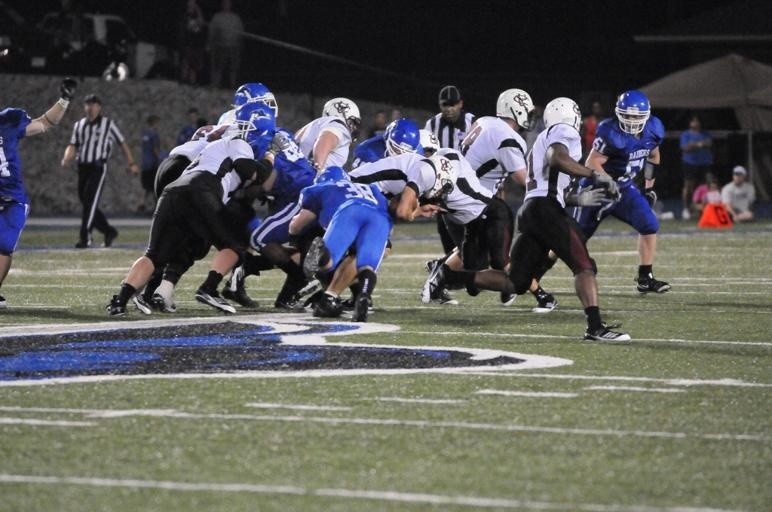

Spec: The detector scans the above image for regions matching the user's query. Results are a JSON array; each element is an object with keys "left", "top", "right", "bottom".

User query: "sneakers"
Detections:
[
  {"left": 421, "top": 263, "right": 448, "bottom": 305},
  {"left": 302, "top": 236, "right": 324, "bottom": 277},
  {"left": 584, "top": 321, "right": 632, "bottom": 342},
  {"left": 500, "top": 291, "right": 516, "bottom": 306},
  {"left": 100, "top": 226, "right": 118, "bottom": 248},
  {"left": 433, "top": 289, "right": 458, "bottom": 306},
  {"left": 532, "top": 293, "right": 559, "bottom": 313},
  {"left": 75, "top": 239, "right": 92, "bottom": 248},
  {"left": 106, "top": 271, "right": 379, "bottom": 322},
  {"left": 636, "top": 272, "right": 670, "bottom": 294}
]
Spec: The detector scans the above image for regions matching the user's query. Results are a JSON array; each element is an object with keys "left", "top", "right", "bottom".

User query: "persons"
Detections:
[
  {"left": 679, "top": 115, "right": 717, "bottom": 220},
  {"left": 107, "top": 82, "right": 671, "bottom": 342},
  {"left": 691, "top": 171, "right": 721, "bottom": 216},
  {"left": 721, "top": 165, "right": 759, "bottom": 222},
  {"left": 0, "top": 77, "right": 77, "bottom": 309},
  {"left": 60, "top": 93, "right": 139, "bottom": 248}
]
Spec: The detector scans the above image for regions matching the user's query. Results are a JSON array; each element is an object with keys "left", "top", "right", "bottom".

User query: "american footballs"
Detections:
[{"left": 388, "top": 194, "right": 418, "bottom": 219}]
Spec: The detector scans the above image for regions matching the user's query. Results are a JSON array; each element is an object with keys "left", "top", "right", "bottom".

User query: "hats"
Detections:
[
  {"left": 439, "top": 84, "right": 461, "bottom": 106},
  {"left": 733, "top": 165, "right": 746, "bottom": 177},
  {"left": 84, "top": 93, "right": 101, "bottom": 104}
]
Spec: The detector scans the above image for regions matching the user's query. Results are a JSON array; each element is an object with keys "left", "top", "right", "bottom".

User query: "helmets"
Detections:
[
  {"left": 496, "top": 87, "right": 538, "bottom": 132},
  {"left": 228, "top": 82, "right": 280, "bottom": 162},
  {"left": 383, "top": 117, "right": 421, "bottom": 158},
  {"left": 422, "top": 155, "right": 458, "bottom": 205},
  {"left": 614, "top": 90, "right": 651, "bottom": 134},
  {"left": 322, "top": 97, "right": 362, "bottom": 134},
  {"left": 543, "top": 95, "right": 582, "bottom": 131},
  {"left": 419, "top": 128, "right": 440, "bottom": 150}
]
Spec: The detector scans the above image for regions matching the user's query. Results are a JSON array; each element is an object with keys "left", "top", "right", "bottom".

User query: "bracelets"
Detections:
[{"left": 58, "top": 98, "right": 71, "bottom": 110}]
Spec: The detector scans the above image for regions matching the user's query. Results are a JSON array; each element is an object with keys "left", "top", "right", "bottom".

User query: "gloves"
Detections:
[
  {"left": 589, "top": 170, "right": 617, "bottom": 198},
  {"left": 256, "top": 184, "right": 280, "bottom": 206},
  {"left": 576, "top": 184, "right": 607, "bottom": 209},
  {"left": 60, "top": 77, "right": 79, "bottom": 102},
  {"left": 268, "top": 132, "right": 290, "bottom": 155},
  {"left": 643, "top": 187, "right": 658, "bottom": 208}
]
